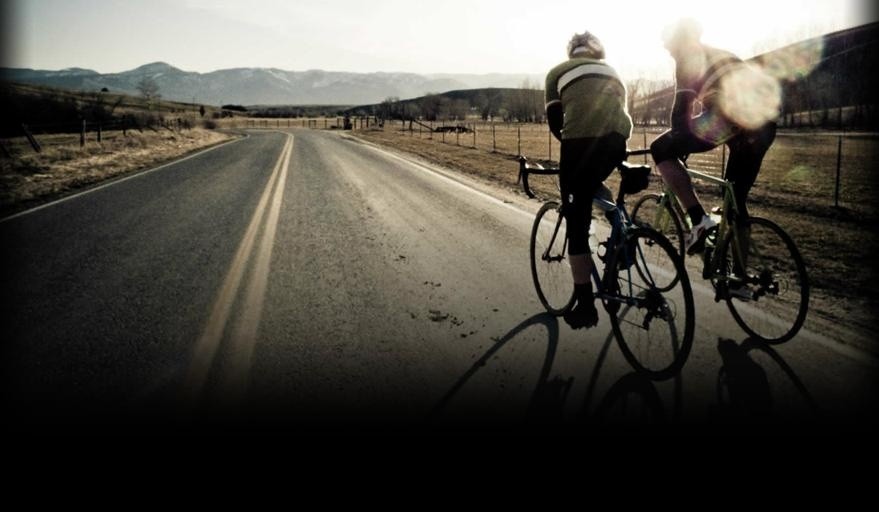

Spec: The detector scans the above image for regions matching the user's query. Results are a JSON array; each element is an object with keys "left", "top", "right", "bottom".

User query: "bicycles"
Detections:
[
  {"left": 630, "top": 148, "right": 810, "bottom": 345},
  {"left": 514, "top": 153, "right": 697, "bottom": 384}
]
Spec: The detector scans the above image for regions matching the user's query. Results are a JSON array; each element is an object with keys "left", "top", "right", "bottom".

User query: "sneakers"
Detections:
[
  {"left": 687, "top": 215, "right": 719, "bottom": 255},
  {"left": 615, "top": 219, "right": 638, "bottom": 270},
  {"left": 714, "top": 273, "right": 751, "bottom": 301},
  {"left": 563, "top": 305, "right": 598, "bottom": 327}
]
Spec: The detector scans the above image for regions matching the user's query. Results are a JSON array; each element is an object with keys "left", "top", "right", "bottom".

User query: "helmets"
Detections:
[{"left": 568, "top": 32, "right": 603, "bottom": 59}]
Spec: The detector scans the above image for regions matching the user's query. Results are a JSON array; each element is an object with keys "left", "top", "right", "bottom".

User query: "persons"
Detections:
[
  {"left": 649, "top": 26, "right": 777, "bottom": 290},
  {"left": 544, "top": 32, "right": 637, "bottom": 330}
]
